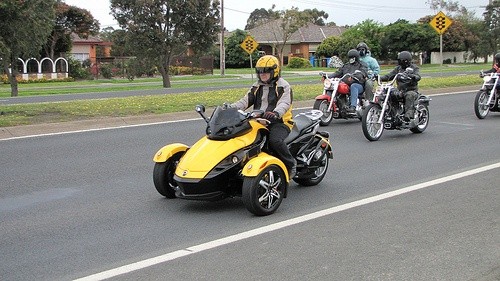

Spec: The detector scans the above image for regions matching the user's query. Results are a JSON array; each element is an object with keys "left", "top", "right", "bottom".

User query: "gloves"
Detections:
[{"left": 262, "top": 112, "right": 279, "bottom": 121}]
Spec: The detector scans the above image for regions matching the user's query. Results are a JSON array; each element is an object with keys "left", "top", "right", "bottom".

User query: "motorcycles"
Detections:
[
  {"left": 473, "top": 68, "right": 500, "bottom": 120},
  {"left": 313, "top": 69, "right": 374, "bottom": 127},
  {"left": 152, "top": 103, "right": 334, "bottom": 216},
  {"left": 362, "top": 67, "right": 433, "bottom": 141}
]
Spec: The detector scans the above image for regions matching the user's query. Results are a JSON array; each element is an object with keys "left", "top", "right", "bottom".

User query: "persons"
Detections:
[
  {"left": 222, "top": 55, "right": 297, "bottom": 179},
  {"left": 356, "top": 42, "right": 381, "bottom": 100},
  {"left": 373, "top": 51, "right": 421, "bottom": 122},
  {"left": 320, "top": 50, "right": 366, "bottom": 112},
  {"left": 479, "top": 53, "right": 500, "bottom": 95}
]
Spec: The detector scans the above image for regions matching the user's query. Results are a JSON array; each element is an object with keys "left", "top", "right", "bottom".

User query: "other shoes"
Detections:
[
  {"left": 350, "top": 106, "right": 356, "bottom": 112},
  {"left": 405, "top": 115, "right": 411, "bottom": 125},
  {"left": 289, "top": 158, "right": 298, "bottom": 180}
]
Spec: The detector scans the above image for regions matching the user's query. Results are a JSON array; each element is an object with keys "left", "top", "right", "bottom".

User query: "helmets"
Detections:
[
  {"left": 495, "top": 53, "right": 500, "bottom": 58},
  {"left": 357, "top": 43, "right": 368, "bottom": 49},
  {"left": 347, "top": 49, "right": 360, "bottom": 61},
  {"left": 255, "top": 55, "right": 280, "bottom": 84},
  {"left": 397, "top": 51, "right": 413, "bottom": 60}
]
